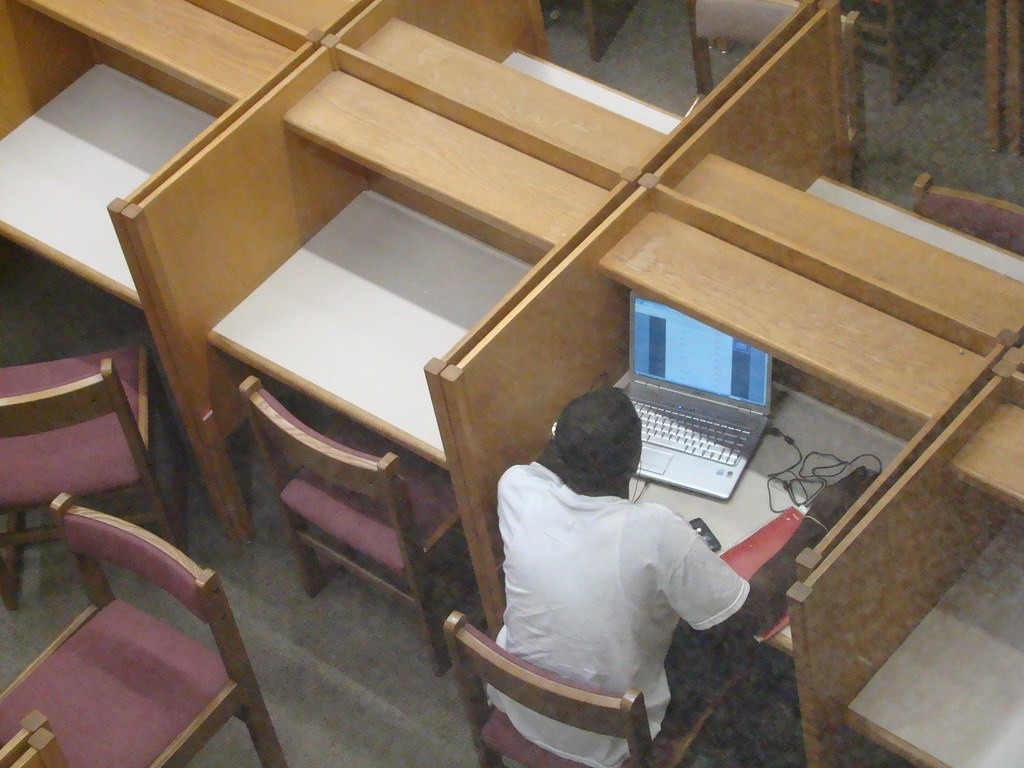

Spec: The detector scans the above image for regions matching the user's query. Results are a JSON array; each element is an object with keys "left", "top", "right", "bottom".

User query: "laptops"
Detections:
[{"left": 621, "top": 284, "right": 772, "bottom": 501}]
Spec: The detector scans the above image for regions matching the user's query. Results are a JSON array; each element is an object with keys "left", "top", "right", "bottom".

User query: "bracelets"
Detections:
[{"left": 805, "top": 516, "right": 828, "bottom": 533}]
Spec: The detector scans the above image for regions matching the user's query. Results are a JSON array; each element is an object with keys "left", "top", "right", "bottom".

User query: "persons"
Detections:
[{"left": 487, "top": 387, "right": 882, "bottom": 768}]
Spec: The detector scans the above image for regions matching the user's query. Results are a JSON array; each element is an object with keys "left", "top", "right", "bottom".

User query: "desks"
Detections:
[{"left": 0, "top": 47, "right": 1024, "bottom": 768}]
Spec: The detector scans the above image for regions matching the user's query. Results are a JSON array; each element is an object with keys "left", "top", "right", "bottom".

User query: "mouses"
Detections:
[{"left": 861, "top": 467, "right": 879, "bottom": 488}]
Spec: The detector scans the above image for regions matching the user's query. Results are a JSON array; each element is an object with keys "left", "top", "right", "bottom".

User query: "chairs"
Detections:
[
  {"left": 0, "top": 493, "right": 288, "bottom": 768},
  {"left": 443, "top": 610, "right": 772, "bottom": 768},
  {"left": 683, "top": 0, "right": 866, "bottom": 193},
  {"left": 239, "top": 373, "right": 458, "bottom": 678},
  {"left": 0, "top": 343, "right": 207, "bottom": 629},
  {"left": 911, "top": 171, "right": 1024, "bottom": 256}
]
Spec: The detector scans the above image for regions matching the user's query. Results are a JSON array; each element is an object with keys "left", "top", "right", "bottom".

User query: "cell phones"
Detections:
[{"left": 689, "top": 518, "right": 721, "bottom": 554}]
